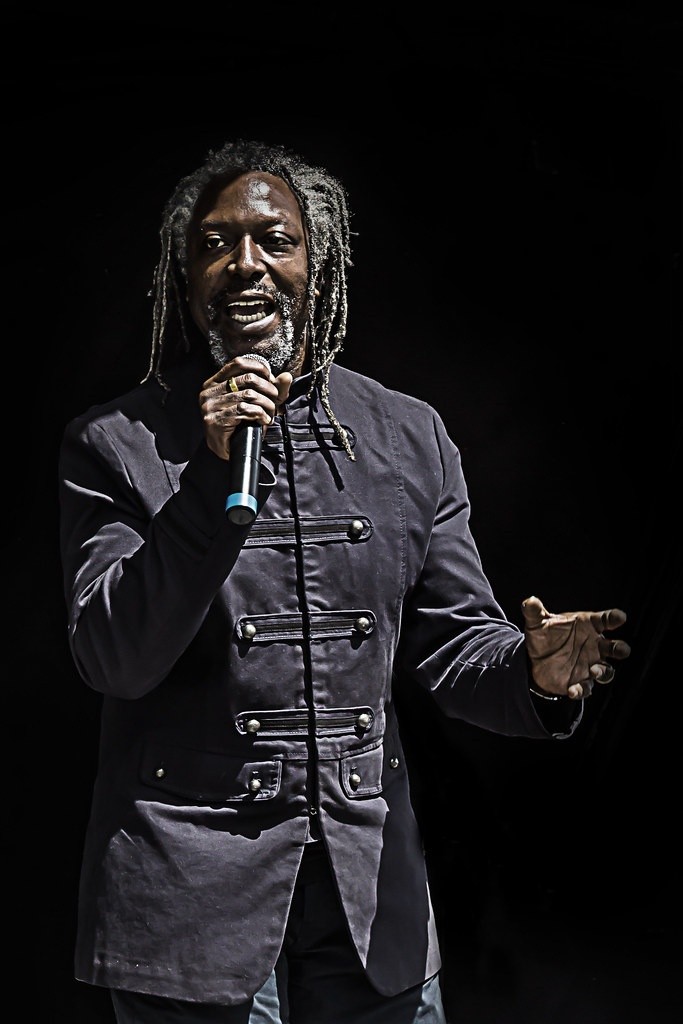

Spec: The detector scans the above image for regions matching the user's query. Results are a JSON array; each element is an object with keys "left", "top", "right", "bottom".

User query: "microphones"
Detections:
[{"left": 224, "top": 353, "right": 272, "bottom": 525}]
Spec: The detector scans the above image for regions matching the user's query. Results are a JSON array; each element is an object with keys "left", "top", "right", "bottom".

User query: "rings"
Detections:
[
  {"left": 591, "top": 660, "right": 616, "bottom": 685},
  {"left": 228, "top": 376, "right": 239, "bottom": 393}
]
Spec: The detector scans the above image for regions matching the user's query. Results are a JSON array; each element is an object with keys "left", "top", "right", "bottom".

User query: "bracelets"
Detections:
[
  {"left": 60, "top": 142, "right": 631, "bottom": 1023},
  {"left": 529, "top": 687, "right": 564, "bottom": 701}
]
